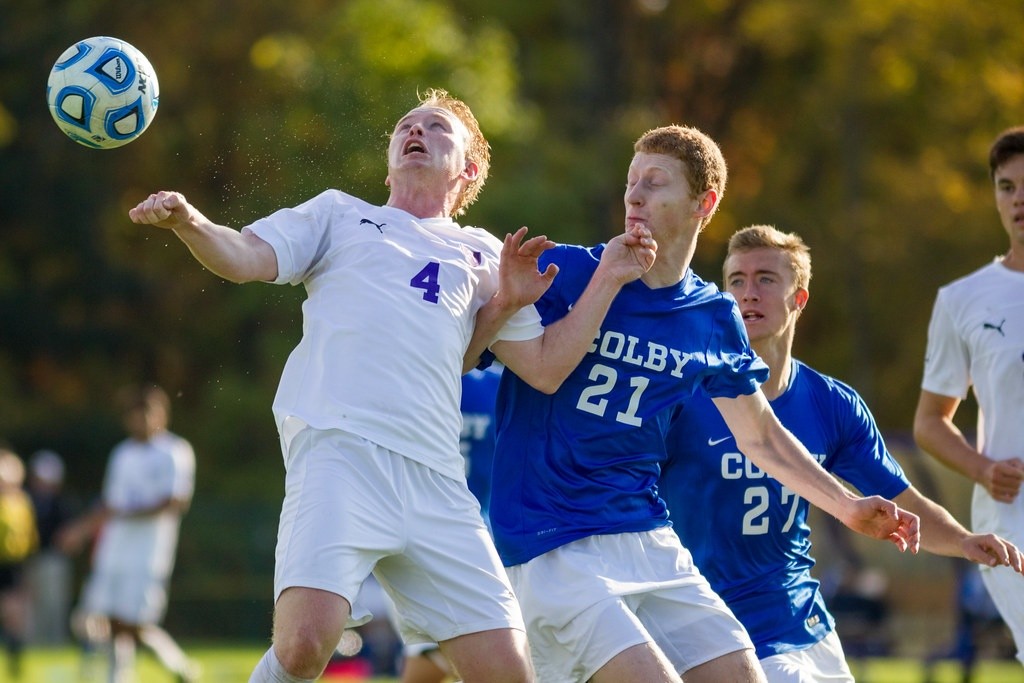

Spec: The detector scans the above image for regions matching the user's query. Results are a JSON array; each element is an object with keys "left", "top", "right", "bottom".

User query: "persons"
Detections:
[
  {"left": 463, "top": 121, "right": 923, "bottom": 683},
  {"left": 913, "top": 122, "right": 1024, "bottom": 683},
  {"left": 384, "top": 356, "right": 506, "bottom": 683},
  {"left": 0, "top": 450, "right": 37, "bottom": 669},
  {"left": 60, "top": 382, "right": 203, "bottom": 683},
  {"left": 129, "top": 84, "right": 658, "bottom": 683},
  {"left": 661, "top": 222, "right": 1024, "bottom": 683}
]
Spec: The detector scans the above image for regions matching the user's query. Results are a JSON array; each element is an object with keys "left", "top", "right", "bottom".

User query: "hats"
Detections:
[{"left": 27, "top": 450, "right": 63, "bottom": 485}]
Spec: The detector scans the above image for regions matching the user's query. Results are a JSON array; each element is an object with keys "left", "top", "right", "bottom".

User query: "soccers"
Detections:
[{"left": 46, "top": 36, "right": 160, "bottom": 150}]
